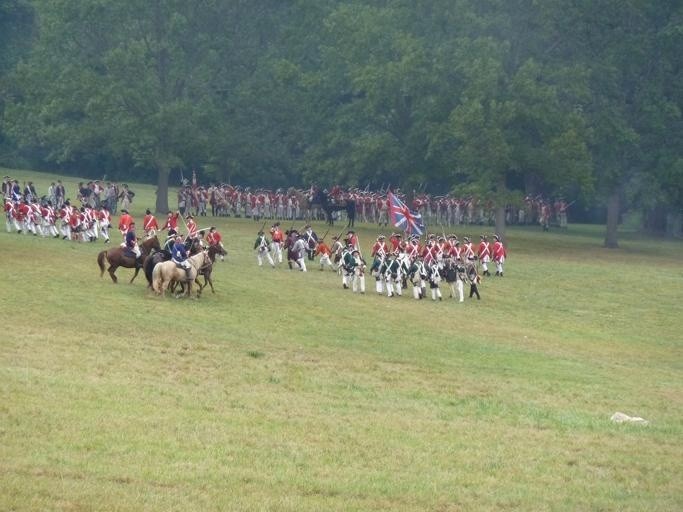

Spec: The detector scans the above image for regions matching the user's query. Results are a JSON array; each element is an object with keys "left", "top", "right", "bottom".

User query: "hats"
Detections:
[
  {"left": 377, "top": 233, "right": 499, "bottom": 246},
  {"left": 1, "top": 175, "right": 127, "bottom": 189},
  {"left": 165, "top": 232, "right": 206, "bottom": 243},
  {"left": 121, "top": 209, "right": 194, "bottom": 217},
  {"left": 209, "top": 227, "right": 217, "bottom": 231},
  {"left": 3, "top": 197, "right": 107, "bottom": 211},
  {"left": 376, "top": 252, "right": 475, "bottom": 264},
  {"left": 185, "top": 185, "right": 288, "bottom": 196},
  {"left": 346, "top": 188, "right": 474, "bottom": 206},
  {"left": 523, "top": 194, "right": 566, "bottom": 207},
  {"left": 258, "top": 222, "right": 360, "bottom": 258}
]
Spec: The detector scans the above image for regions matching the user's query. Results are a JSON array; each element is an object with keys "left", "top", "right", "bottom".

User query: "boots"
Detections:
[
  {"left": 135, "top": 258, "right": 142, "bottom": 268},
  {"left": 201, "top": 212, "right": 324, "bottom": 220},
  {"left": 483, "top": 269, "right": 504, "bottom": 276},
  {"left": 185, "top": 268, "right": 193, "bottom": 280},
  {"left": 259, "top": 258, "right": 337, "bottom": 272}
]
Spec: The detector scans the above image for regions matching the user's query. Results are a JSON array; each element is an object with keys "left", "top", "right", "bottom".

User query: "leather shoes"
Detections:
[
  {"left": 16, "top": 229, "right": 109, "bottom": 243},
  {"left": 342, "top": 284, "right": 480, "bottom": 302},
  {"left": 424, "top": 221, "right": 569, "bottom": 226}
]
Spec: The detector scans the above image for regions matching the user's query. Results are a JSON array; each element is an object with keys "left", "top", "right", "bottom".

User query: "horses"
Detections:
[
  {"left": 286, "top": 184, "right": 329, "bottom": 224},
  {"left": 97, "top": 234, "right": 161, "bottom": 284},
  {"left": 312, "top": 187, "right": 356, "bottom": 228},
  {"left": 143, "top": 242, "right": 228, "bottom": 299}
]
